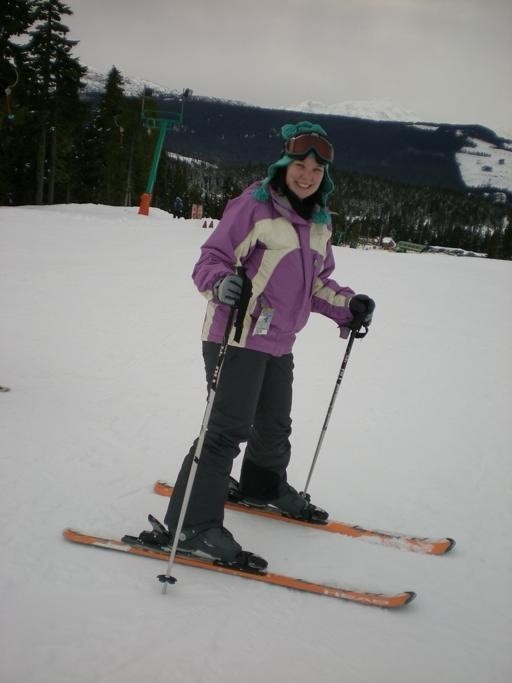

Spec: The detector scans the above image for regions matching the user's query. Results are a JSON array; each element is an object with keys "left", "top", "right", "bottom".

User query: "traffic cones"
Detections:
[{"left": 201, "top": 220, "right": 213, "bottom": 228}]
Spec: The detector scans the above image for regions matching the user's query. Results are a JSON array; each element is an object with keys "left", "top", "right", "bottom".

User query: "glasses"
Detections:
[{"left": 283, "top": 130, "right": 334, "bottom": 163}]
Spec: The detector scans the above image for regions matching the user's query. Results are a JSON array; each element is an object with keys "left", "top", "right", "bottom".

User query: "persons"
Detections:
[
  {"left": 171, "top": 196, "right": 184, "bottom": 219},
  {"left": 164, "top": 119, "right": 374, "bottom": 571}
]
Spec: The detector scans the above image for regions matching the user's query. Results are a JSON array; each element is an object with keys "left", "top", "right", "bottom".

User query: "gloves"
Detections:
[
  {"left": 210, "top": 271, "right": 246, "bottom": 309},
  {"left": 348, "top": 293, "right": 376, "bottom": 328}
]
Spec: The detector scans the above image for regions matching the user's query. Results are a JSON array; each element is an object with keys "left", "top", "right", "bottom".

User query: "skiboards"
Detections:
[{"left": 63, "top": 481, "right": 454, "bottom": 609}]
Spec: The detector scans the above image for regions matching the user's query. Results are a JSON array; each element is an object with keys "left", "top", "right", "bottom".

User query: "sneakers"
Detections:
[
  {"left": 238, "top": 473, "right": 309, "bottom": 517},
  {"left": 164, "top": 522, "right": 243, "bottom": 564}
]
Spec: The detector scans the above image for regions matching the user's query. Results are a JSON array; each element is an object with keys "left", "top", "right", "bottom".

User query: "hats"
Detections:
[{"left": 251, "top": 119, "right": 339, "bottom": 226}]
